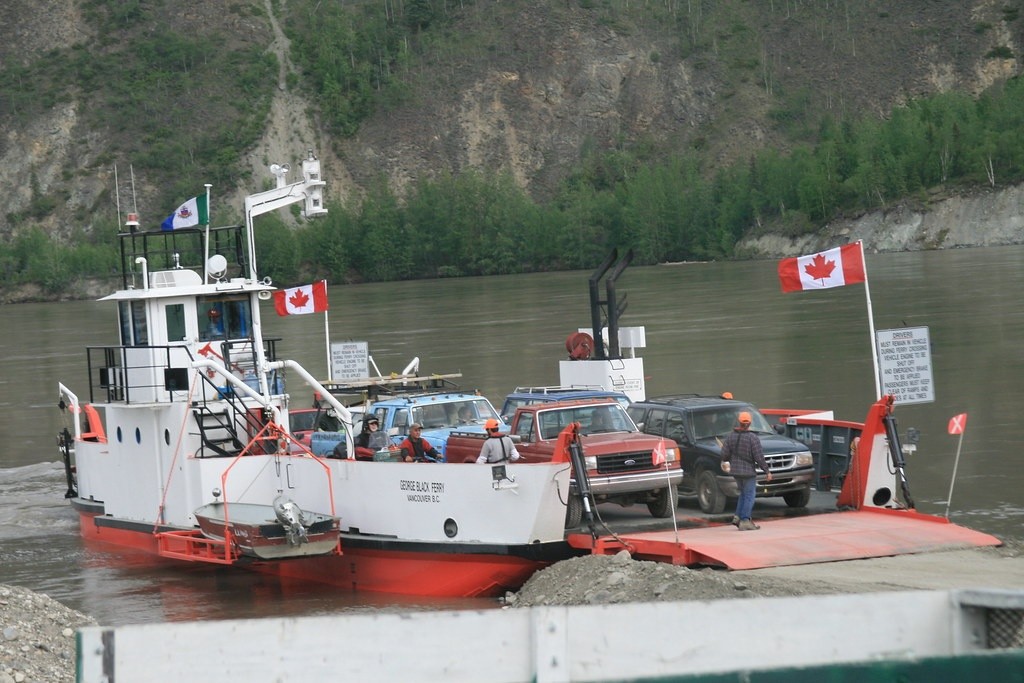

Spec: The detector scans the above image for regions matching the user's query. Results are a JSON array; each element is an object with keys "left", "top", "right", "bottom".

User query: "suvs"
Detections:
[
  {"left": 492, "top": 384, "right": 637, "bottom": 437},
  {"left": 619, "top": 393, "right": 816, "bottom": 515}
]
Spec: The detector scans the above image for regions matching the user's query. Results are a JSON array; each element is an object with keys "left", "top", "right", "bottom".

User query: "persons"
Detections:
[
  {"left": 204, "top": 308, "right": 224, "bottom": 336},
  {"left": 348, "top": 403, "right": 520, "bottom": 465},
  {"left": 584, "top": 409, "right": 612, "bottom": 434},
  {"left": 718, "top": 412, "right": 773, "bottom": 531}
]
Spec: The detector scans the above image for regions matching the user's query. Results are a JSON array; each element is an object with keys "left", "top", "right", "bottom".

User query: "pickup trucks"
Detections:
[
  {"left": 243, "top": 391, "right": 517, "bottom": 461},
  {"left": 444, "top": 399, "right": 683, "bottom": 530}
]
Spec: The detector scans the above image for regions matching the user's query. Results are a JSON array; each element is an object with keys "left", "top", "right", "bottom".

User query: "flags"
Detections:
[
  {"left": 777, "top": 241, "right": 865, "bottom": 294},
  {"left": 272, "top": 280, "right": 330, "bottom": 318},
  {"left": 948, "top": 413, "right": 967, "bottom": 435},
  {"left": 649, "top": 439, "right": 668, "bottom": 466},
  {"left": 161, "top": 193, "right": 209, "bottom": 231}
]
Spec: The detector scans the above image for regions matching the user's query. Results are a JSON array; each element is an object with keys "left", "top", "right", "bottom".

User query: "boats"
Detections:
[
  {"left": 43, "top": 151, "right": 580, "bottom": 597},
  {"left": 190, "top": 501, "right": 339, "bottom": 559}
]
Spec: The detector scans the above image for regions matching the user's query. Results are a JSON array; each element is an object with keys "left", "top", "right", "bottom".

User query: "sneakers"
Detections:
[
  {"left": 739, "top": 520, "right": 760, "bottom": 531},
  {"left": 731, "top": 515, "right": 740, "bottom": 528}
]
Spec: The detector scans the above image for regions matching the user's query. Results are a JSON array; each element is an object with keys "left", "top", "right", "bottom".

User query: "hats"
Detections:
[
  {"left": 737, "top": 412, "right": 751, "bottom": 424},
  {"left": 409, "top": 423, "right": 423, "bottom": 432},
  {"left": 483, "top": 419, "right": 499, "bottom": 428},
  {"left": 368, "top": 419, "right": 377, "bottom": 424}
]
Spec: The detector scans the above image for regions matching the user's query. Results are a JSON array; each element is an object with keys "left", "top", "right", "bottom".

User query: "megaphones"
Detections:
[{"left": 258, "top": 291, "right": 271, "bottom": 300}]
